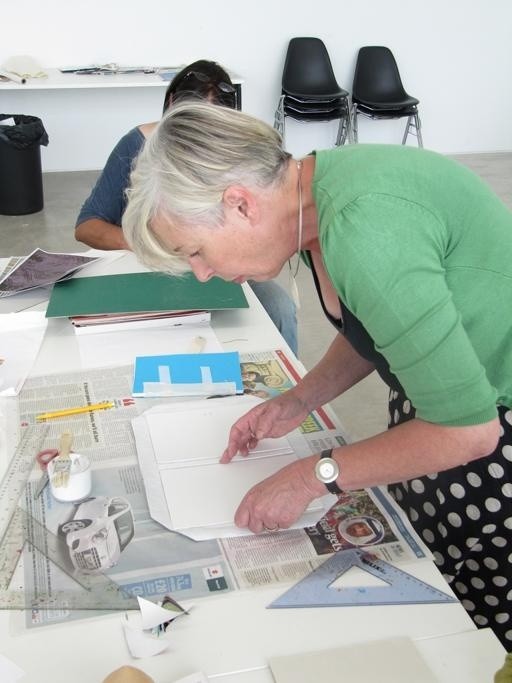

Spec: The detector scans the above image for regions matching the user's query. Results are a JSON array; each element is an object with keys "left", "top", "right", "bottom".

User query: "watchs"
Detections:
[{"left": 312, "top": 447, "right": 344, "bottom": 497}]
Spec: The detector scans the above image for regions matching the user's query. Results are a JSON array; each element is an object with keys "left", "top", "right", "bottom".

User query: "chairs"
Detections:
[{"left": 269, "top": 33, "right": 434, "bottom": 151}]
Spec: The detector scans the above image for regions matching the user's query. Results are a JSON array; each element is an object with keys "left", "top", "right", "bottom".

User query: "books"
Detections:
[{"left": 70, "top": 310, "right": 211, "bottom": 336}]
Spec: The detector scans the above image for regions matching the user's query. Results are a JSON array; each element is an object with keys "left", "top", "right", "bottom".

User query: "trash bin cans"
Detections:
[{"left": 0, "top": 114, "right": 49, "bottom": 216}]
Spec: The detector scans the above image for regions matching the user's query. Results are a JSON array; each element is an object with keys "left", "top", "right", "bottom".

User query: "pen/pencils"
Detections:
[{"left": 36, "top": 403, "right": 115, "bottom": 420}]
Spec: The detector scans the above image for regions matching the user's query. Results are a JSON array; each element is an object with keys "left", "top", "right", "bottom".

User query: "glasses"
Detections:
[{"left": 173, "top": 70, "right": 237, "bottom": 95}]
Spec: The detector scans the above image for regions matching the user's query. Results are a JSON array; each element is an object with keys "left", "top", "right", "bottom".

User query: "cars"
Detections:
[{"left": 59, "top": 494, "right": 138, "bottom": 576}]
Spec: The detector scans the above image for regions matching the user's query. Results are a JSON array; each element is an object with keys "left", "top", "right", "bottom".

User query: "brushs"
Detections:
[{"left": 52, "top": 431, "right": 74, "bottom": 488}]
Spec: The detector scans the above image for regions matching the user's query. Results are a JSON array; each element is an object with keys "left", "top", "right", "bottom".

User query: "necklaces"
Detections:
[{"left": 289, "top": 159, "right": 303, "bottom": 308}]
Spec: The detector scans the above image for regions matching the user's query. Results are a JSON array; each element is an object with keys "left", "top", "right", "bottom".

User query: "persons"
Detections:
[
  {"left": 337, "top": 515, "right": 384, "bottom": 546},
  {"left": 241, "top": 366, "right": 269, "bottom": 398},
  {"left": 120, "top": 100, "right": 512, "bottom": 654},
  {"left": 74, "top": 60, "right": 302, "bottom": 360}
]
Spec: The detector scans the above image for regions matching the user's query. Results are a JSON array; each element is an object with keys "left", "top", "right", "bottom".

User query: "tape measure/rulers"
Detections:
[
  {"left": 265, "top": 541, "right": 460, "bottom": 609},
  {"left": 0, "top": 504, "right": 141, "bottom": 610},
  {"left": 0, "top": 424, "right": 50, "bottom": 546}
]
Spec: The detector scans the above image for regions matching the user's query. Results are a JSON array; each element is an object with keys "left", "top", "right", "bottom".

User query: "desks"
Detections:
[
  {"left": 0, "top": 68, "right": 245, "bottom": 114},
  {"left": 1, "top": 252, "right": 511, "bottom": 681}
]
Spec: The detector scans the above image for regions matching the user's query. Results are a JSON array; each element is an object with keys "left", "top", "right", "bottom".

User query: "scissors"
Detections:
[{"left": 36, "top": 448, "right": 74, "bottom": 498}]
